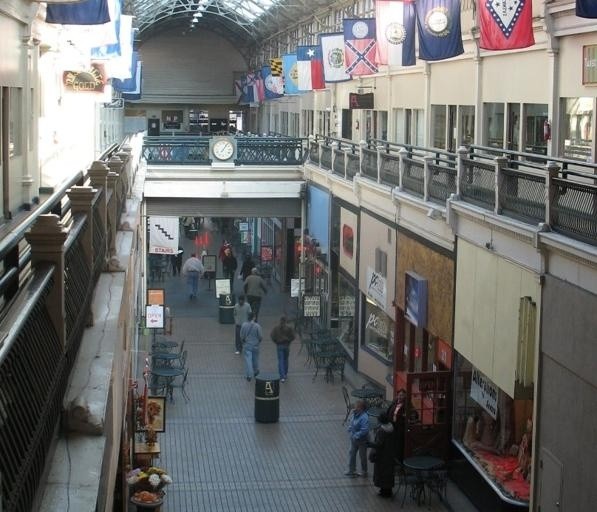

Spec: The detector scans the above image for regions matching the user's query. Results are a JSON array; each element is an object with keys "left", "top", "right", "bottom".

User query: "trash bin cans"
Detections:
[
  {"left": 255, "top": 373, "right": 280, "bottom": 423},
  {"left": 219, "top": 293, "right": 235, "bottom": 324}
]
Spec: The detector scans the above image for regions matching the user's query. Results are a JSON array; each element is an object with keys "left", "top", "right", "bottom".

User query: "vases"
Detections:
[{"left": 130, "top": 495, "right": 164, "bottom": 512}]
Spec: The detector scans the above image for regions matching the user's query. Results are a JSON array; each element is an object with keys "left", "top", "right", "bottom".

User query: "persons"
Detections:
[
  {"left": 344, "top": 386, "right": 410, "bottom": 499},
  {"left": 270, "top": 314, "right": 295, "bottom": 384},
  {"left": 218, "top": 238, "right": 265, "bottom": 355},
  {"left": 480, "top": 409, "right": 498, "bottom": 446},
  {"left": 239, "top": 311, "right": 263, "bottom": 382},
  {"left": 147, "top": 244, "right": 184, "bottom": 283},
  {"left": 180, "top": 216, "right": 205, "bottom": 237},
  {"left": 181, "top": 253, "right": 204, "bottom": 300}
]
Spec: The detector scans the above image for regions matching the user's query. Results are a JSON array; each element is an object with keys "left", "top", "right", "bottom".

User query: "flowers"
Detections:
[{"left": 124, "top": 464, "right": 173, "bottom": 504}]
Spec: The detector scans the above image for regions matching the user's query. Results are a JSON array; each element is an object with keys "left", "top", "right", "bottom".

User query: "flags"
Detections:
[
  {"left": 44, "top": 0, "right": 144, "bottom": 102},
  {"left": 575, "top": 1, "right": 597, "bottom": 18},
  {"left": 476, "top": 0, "right": 536, "bottom": 51},
  {"left": 234, "top": 0, "right": 464, "bottom": 106}
]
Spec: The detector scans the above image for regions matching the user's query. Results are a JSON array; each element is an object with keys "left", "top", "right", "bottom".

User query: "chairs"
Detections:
[
  {"left": 342, "top": 384, "right": 448, "bottom": 511},
  {"left": 148, "top": 340, "right": 191, "bottom": 404},
  {"left": 295, "top": 321, "right": 347, "bottom": 383}
]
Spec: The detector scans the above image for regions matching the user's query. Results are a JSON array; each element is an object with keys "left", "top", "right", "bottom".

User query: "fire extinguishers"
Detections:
[{"left": 544, "top": 119, "right": 550, "bottom": 142}]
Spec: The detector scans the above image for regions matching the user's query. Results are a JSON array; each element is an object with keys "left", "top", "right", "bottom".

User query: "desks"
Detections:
[{"left": 134, "top": 442, "right": 160, "bottom": 466}]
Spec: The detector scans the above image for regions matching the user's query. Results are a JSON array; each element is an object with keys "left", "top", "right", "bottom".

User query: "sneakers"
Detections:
[
  {"left": 281, "top": 378, "right": 286, "bottom": 382},
  {"left": 235, "top": 351, "right": 241, "bottom": 355},
  {"left": 344, "top": 470, "right": 367, "bottom": 480},
  {"left": 244, "top": 369, "right": 259, "bottom": 382}
]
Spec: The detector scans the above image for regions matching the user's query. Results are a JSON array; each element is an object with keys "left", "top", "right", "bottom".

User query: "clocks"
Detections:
[{"left": 212, "top": 138, "right": 235, "bottom": 161}]
